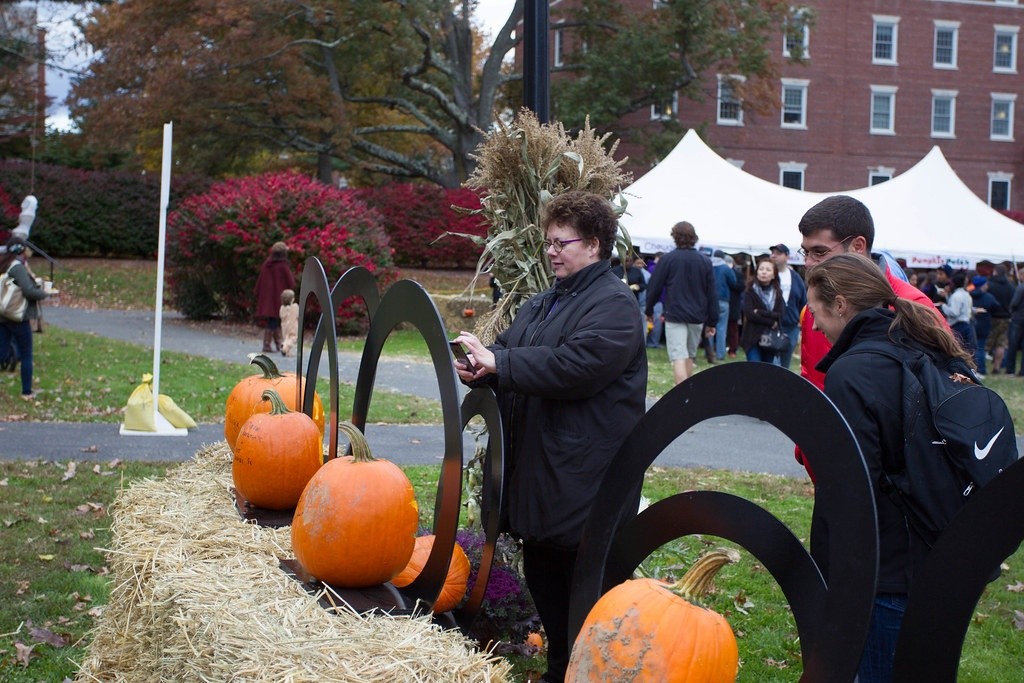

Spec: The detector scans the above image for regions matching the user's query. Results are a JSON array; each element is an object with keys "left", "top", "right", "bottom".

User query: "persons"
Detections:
[
  {"left": 0, "top": 236, "right": 48, "bottom": 401},
  {"left": 253, "top": 242, "right": 296, "bottom": 352},
  {"left": 278, "top": 288, "right": 299, "bottom": 356},
  {"left": 798, "top": 257, "right": 980, "bottom": 683},
  {"left": 452, "top": 192, "right": 650, "bottom": 683},
  {"left": 794, "top": 194, "right": 962, "bottom": 590},
  {"left": 625, "top": 223, "right": 1024, "bottom": 386}
]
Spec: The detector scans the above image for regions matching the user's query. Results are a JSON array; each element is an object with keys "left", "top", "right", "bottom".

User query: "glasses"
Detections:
[
  {"left": 797, "top": 235, "right": 856, "bottom": 260},
  {"left": 542, "top": 238, "right": 583, "bottom": 253}
]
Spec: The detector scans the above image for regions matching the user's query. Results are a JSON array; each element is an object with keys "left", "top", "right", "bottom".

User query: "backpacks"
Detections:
[{"left": 838, "top": 339, "right": 1024, "bottom": 570}]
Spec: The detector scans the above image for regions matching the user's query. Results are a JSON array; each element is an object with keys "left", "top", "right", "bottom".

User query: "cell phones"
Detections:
[{"left": 449, "top": 341, "right": 477, "bottom": 376}]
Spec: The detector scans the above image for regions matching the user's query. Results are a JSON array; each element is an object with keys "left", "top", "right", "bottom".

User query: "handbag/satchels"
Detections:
[
  {"left": 759, "top": 319, "right": 790, "bottom": 353},
  {"left": 0, "top": 260, "right": 28, "bottom": 322}
]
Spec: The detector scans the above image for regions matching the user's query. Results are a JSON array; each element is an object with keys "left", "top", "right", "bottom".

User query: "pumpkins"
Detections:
[
  {"left": 526, "top": 632, "right": 542, "bottom": 652},
  {"left": 224, "top": 354, "right": 470, "bottom": 616},
  {"left": 564, "top": 553, "right": 738, "bottom": 683}
]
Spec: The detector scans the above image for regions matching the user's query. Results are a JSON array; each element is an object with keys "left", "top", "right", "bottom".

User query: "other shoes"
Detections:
[
  {"left": 974, "top": 368, "right": 1024, "bottom": 380},
  {"left": 707, "top": 350, "right": 736, "bottom": 363},
  {"left": 23, "top": 392, "right": 36, "bottom": 399}
]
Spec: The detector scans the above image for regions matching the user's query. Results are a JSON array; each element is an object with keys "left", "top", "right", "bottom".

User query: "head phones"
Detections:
[{"left": 9, "top": 244, "right": 25, "bottom": 254}]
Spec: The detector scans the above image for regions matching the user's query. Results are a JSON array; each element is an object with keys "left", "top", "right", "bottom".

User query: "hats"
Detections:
[
  {"left": 972, "top": 274, "right": 987, "bottom": 288},
  {"left": 769, "top": 244, "right": 790, "bottom": 255},
  {"left": 937, "top": 265, "right": 952, "bottom": 278},
  {"left": 724, "top": 256, "right": 733, "bottom": 269}
]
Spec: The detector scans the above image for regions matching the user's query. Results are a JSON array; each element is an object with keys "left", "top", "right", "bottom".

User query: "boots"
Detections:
[{"left": 263, "top": 327, "right": 283, "bottom": 352}]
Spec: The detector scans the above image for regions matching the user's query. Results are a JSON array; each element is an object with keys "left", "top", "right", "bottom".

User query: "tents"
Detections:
[{"left": 608, "top": 128, "right": 1024, "bottom": 271}]
